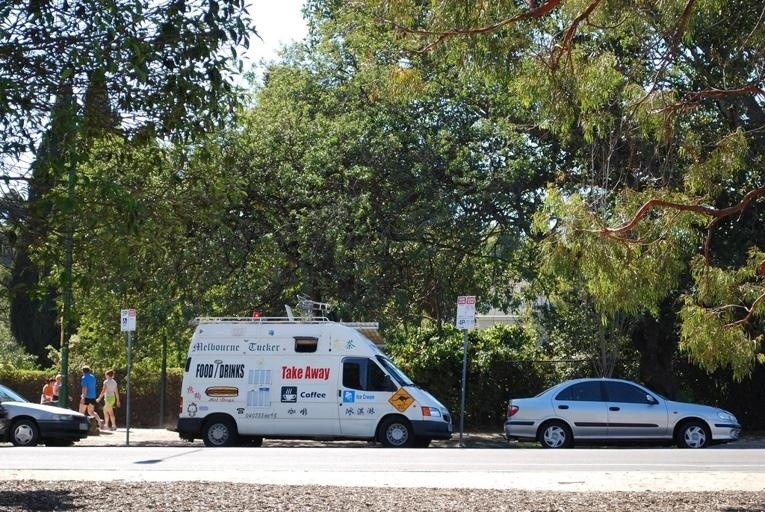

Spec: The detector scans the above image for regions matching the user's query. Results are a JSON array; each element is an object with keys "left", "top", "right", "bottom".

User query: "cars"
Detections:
[
  {"left": 0, "top": 384, "right": 93, "bottom": 447},
  {"left": 502, "top": 376, "right": 742, "bottom": 449}
]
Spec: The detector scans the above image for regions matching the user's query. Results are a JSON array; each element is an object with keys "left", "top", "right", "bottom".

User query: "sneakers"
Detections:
[
  {"left": 110, "top": 427, "right": 116, "bottom": 430},
  {"left": 99, "top": 420, "right": 104, "bottom": 430}
]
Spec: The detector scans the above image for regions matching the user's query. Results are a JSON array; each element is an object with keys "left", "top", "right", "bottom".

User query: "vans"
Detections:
[{"left": 177, "top": 291, "right": 453, "bottom": 449}]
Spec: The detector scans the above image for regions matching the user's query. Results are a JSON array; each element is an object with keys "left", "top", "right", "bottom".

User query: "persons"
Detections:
[
  {"left": 53, "top": 374, "right": 61, "bottom": 400},
  {"left": 96, "top": 369, "right": 121, "bottom": 431},
  {"left": 40, "top": 377, "right": 56, "bottom": 404},
  {"left": 78, "top": 365, "right": 105, "bottom": 429}
]
